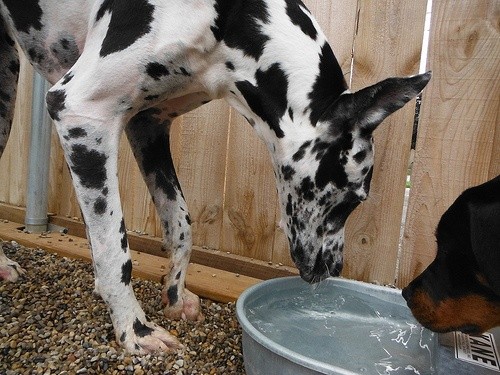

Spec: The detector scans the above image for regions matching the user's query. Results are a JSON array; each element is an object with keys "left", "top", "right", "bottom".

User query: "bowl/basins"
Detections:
[{"left": 237, "top": 267, "right": 500, "bottom": 374}]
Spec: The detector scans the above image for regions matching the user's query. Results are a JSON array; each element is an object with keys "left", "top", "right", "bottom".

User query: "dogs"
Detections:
[
  {"left": 402, "top": 174, "right": 500, "bottom": 337},
  {"left": 1, "top": 0, "right": 431, "bottom": 354}
]
